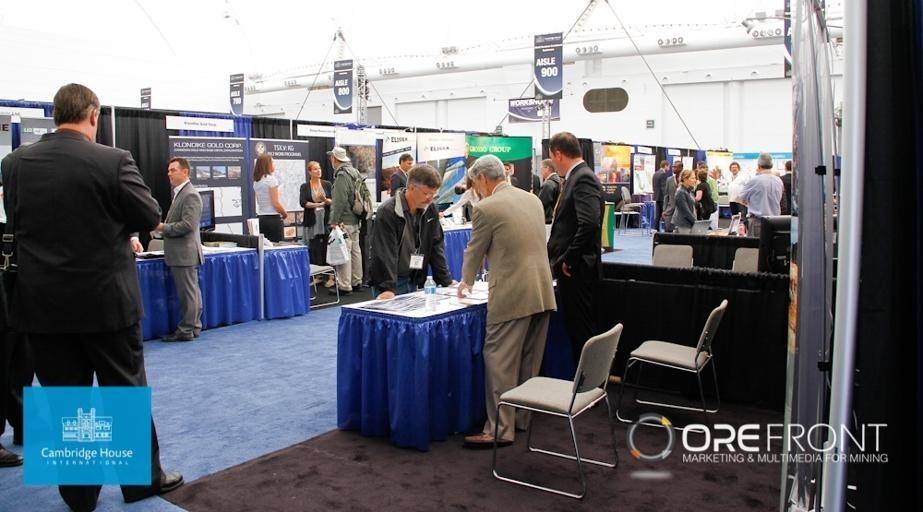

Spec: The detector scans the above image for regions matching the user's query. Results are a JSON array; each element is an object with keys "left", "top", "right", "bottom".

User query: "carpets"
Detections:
[{"left": 157, "top": 389, "right": 781, "bottom": 512}]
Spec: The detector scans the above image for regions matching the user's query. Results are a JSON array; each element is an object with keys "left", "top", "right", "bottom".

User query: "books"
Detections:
[{"left": 203, "top": 241, "right": 238, "bottom": 249}]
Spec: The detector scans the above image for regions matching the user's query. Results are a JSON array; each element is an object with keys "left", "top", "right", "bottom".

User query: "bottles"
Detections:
[{"left": 423, "top": 275, "right": 437, "bottom": 312}]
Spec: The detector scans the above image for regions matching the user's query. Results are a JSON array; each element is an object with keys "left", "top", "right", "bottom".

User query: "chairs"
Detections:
[
  {"left": 493, "top": 323, "right": 625, "bottom": 499},
  {"left": 310, "top": 265, "right": 339, "bottom": 308},
  {"left": 617, "top": 299, "right": 730, "bottom": 432},
  {"left": 653, "top": 245, "right": 693, "bottom": 267},
  {"left": 731, "top": 247, "right": 760, "bottom": 271},
  {"left": 429, "top": 222, "right": 491, "bottom": 275},
  {"left": 618, "top": 186, "right": 645, "bottom": 236}
]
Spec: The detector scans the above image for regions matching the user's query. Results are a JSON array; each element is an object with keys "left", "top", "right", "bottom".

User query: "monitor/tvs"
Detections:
[
  {"left": 758, "top": 215, "right": 790, "bottom": 275},
  {"left": 197, "top": 190, "right": 215, "bottom": 231}
]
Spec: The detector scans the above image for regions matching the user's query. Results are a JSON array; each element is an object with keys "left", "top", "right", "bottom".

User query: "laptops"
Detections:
[
  {"left": 728, "top": 215, "right": 740, "bottom": 235},
  {"left": 689, "top": 220, "right": 712, "bottom": 234}
]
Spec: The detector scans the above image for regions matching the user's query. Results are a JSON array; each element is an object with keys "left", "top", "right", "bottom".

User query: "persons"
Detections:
[
  {"left": 387, "top": 152, "right": 413, "bottom": 197},
  {"left": 458, "top": 154, "right": 558, "bottom": 444},
  {"left": 652, "top": 152, "right": 792, "bottom": 236},
  {"left": 547, "top": 132, "right": 605, "bottom": 360},
  {"left": 439, "top": 157, "right": 487, "bottom": 283},
  {"left": 503, "top": 162, "right": 522, "bottom": 187},
  {"left": 252, "top": 152, "right": 286, "bottom": 243},
  {"left": 298, "top": 161, "right": 334, "bottom": 287},
  {"left": 372, "top": 164, "right": 458, "bottom": 300},
  {"left": 154, "top": 159, "right": 203, "bottom": 340},
  {"left": 1, "top": 362, "right": 33, "bottom": 467},
  {"left": 326, "top": 145, "right": 371, "bottom": 296},
  {"left": 128, "top": 230, "right": 151, "bottom": 254},
  {"left": 0, "top": 83, "right": 182, "bottom": 512},
  {"left": 535, "top": 159, "right": 560, "bottom": 223}
]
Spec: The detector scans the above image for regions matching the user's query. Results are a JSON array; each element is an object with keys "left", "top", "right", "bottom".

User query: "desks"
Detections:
[
  {"left": 339, "top": 281, "right": 559, "bottom": 451},
  {"left": 264, "top": 244, "right": 310, "bottom": 320},
  {"left": 135, "top": 246, "right": 260, "bottom": 340}
]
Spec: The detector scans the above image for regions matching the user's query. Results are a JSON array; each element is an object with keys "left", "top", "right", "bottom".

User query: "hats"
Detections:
[{"left": 326, "top": 147, "right": 352, "bottom": 162}]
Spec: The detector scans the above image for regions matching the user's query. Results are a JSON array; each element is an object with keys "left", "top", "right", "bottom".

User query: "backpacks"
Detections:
[{"left": 339, "top": 171, "right": 372, "bottom": 220}]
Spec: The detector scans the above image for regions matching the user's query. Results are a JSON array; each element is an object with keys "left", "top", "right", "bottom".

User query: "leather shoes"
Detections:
[
  {"left": 464, "top": 435, "right": 514, "bottom": 443},
  {"left": 1, "top": 444, "right": 23, "bottom": 467},
  {"left": 310, "top": 274, "right": 363, "bottom": 295},
  {"left": 161, "top": 471, "right": 184, "bottom": 491},
  {"left": 161, "top": 334, "right": 194, "bottom": 342}
]
